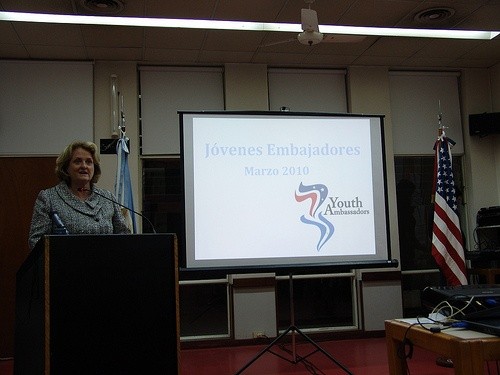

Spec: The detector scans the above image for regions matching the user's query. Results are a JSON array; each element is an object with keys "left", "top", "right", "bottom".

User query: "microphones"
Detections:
[
  {"left": 77, "top": 187, "right": 156, "bottom": 233},
  {"left": 49, "top": 210, "right": 68, "bottom": 234}
]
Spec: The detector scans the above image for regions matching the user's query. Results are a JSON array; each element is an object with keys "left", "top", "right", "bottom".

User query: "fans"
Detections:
[{"left": 254, "top": 0, "right": 367, "bottom": 49}]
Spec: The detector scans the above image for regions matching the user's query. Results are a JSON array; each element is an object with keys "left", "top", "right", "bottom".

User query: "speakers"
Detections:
[{"left": 468, "top": 112, "right": 500, "bottom": 136}]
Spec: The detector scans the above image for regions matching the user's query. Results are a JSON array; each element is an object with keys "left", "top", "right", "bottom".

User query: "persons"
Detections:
[{"left": 28, "top": 140, "right": 131, "bottom": 251}]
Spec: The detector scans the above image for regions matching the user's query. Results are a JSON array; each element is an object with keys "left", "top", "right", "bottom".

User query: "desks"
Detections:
[{"left": 385, "top": 318, "right": 500, "bottom": 375}]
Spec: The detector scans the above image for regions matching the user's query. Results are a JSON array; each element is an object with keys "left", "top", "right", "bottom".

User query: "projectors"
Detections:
[{"left": 420, "top": 284, "right": 500, "bottom": 320}]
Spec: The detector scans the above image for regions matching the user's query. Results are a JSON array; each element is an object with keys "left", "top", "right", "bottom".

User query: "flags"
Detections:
[
  {"left": 431, "top": 135, "right": 468, "bottom": 286},
  {"left": 114, "top": 139, "right": 138, "bottom": 233}
]
